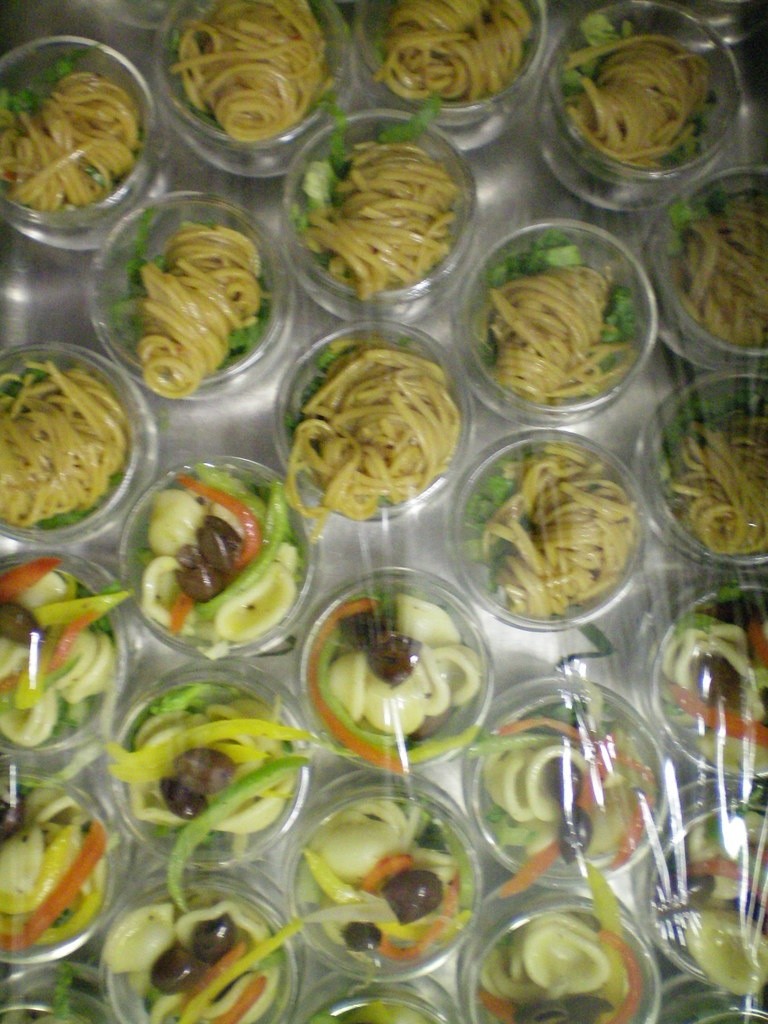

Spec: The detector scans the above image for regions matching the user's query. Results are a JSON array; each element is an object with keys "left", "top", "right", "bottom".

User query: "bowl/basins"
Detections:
[
  {"left": 639, "top": 795, "right": 768, "bottom": 1001},
  {"left": 84, "top": 190, "right": 297, "bottom": 400},
  {"left": 662, "top": 987, "right": 768, "bottom": 1024},
  {"left": 0, "top": 552, "right": 138, "bottom": 760},
  {"left": 0, "top": 34, "right": 175, "bottom": 256},
  {"left": 636, "top": 366, "right": 768, "bottom": 571},
  {"left": 346, "top": 0, "right": 550, "bottom": 152},
  {"left": 642, "top": 163, "right": 768, "bottom": 378},
  {"left": 0, "top": 342, "right": 161, "bottom": 555},
  {"left": 540, "top": 0, "right": 745, "bottom": 209},
  {"left": 65, "top": 96, "right": 685, "bottom": 1024},
  {"left": 151, "top": 0, "right": 355, "bottom": 177},
  {"left": 0, "top": 963, "right": 107, "bottom": 1024},
  {"left": 636, "top": 582, "right": 768, "bottom": 783},
  {"left": 0, "top": 763, "right": 123, "bottom": 967}
]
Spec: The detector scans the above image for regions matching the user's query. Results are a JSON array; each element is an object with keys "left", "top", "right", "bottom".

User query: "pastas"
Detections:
[{"left": 0, "top": 0, "right": 767, "bottom": 626}]
